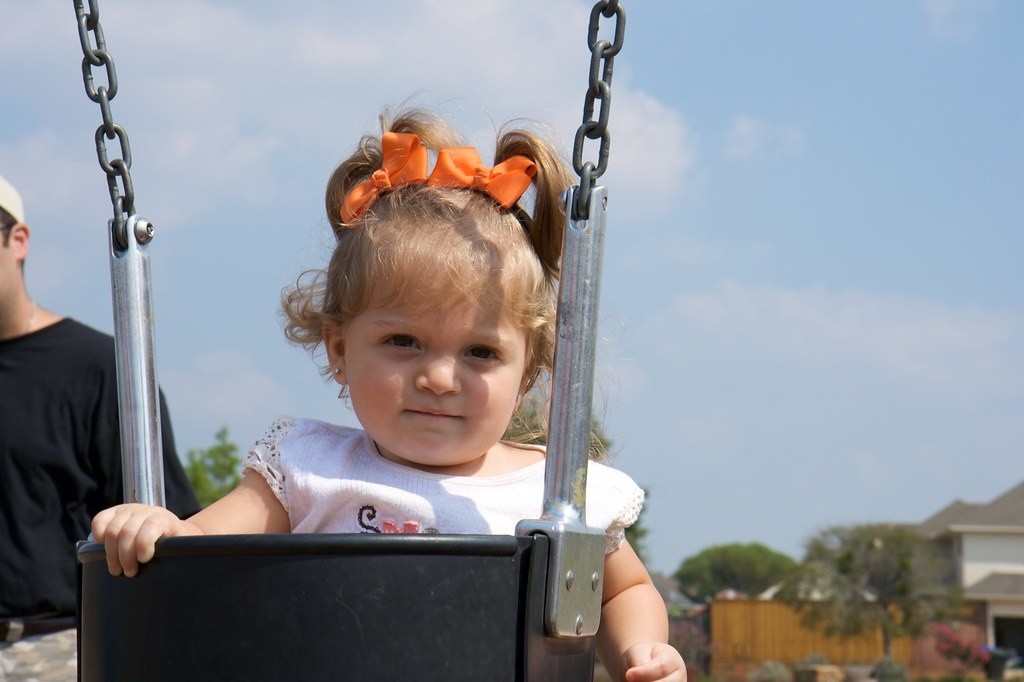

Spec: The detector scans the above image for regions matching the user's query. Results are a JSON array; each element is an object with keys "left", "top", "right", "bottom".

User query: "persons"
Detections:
[
  {"left": 0, "top": 173, "right": 201, "bottom": 682},
  {"left": 88, "top": 105, "right": 688, "bottom": 682}
]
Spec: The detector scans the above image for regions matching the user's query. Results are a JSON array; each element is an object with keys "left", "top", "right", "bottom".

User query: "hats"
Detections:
[{"left": 0, "top": 176, "right": 25, "bottom": 224}]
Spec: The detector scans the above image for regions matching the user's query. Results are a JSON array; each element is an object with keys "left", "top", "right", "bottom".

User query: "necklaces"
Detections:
[{"left": 27, "top": 300, "right": 35, "bottom": 334}]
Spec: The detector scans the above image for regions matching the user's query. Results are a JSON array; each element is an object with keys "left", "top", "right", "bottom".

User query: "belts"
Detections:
[{"left": 0, "top": 614, "right": 77, "bottom": 644}]
